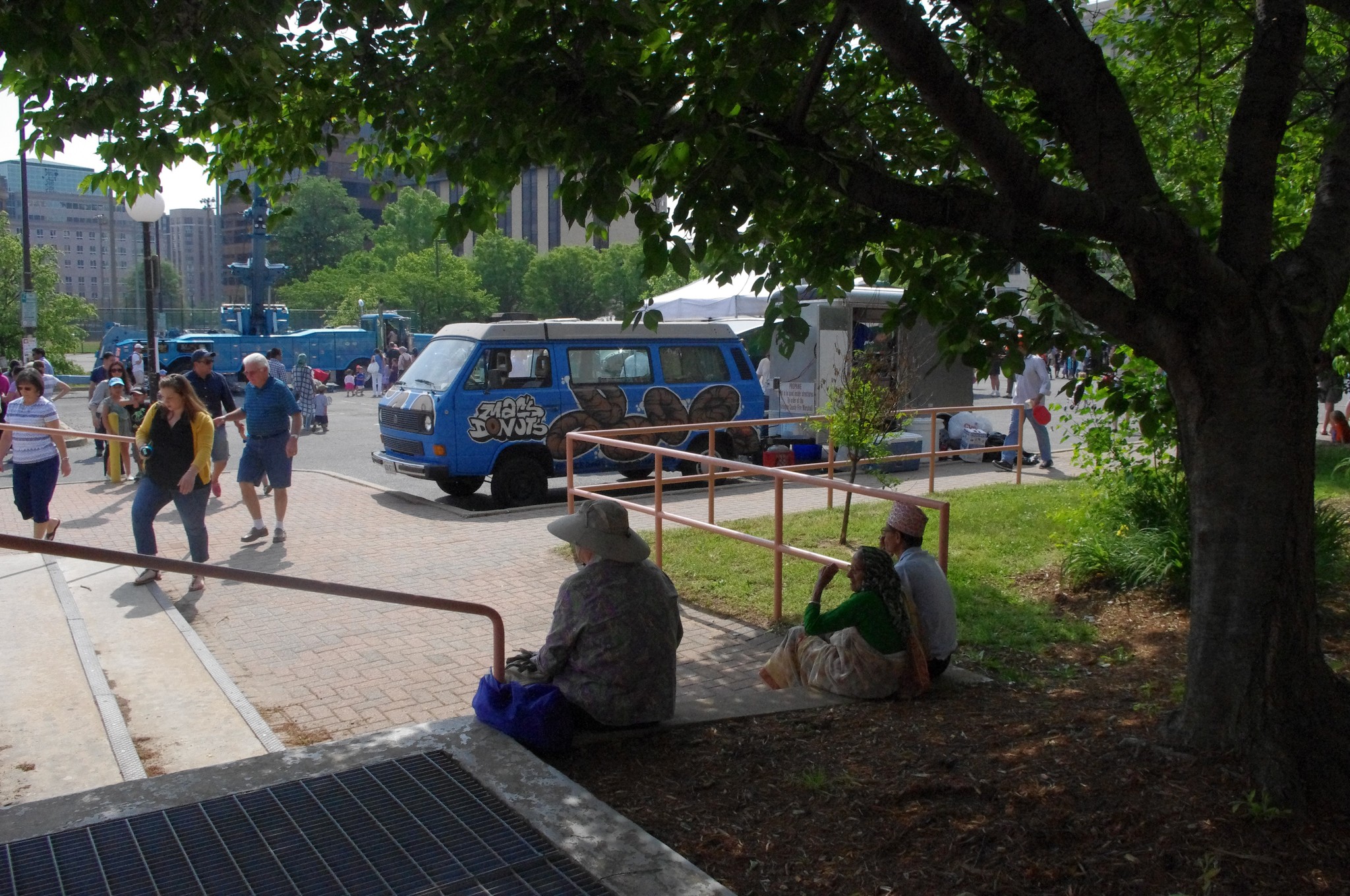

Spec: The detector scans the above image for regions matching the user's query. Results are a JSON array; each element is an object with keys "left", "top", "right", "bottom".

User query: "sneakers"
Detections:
[
  {"left": 990, "top": 391, "right": 1000, "bottom": 396},
  {"left": 263, "top": 485, "right": 273, "bottom": 494},
  {"left": 210, "top": 473, "right": 221, "bottom": 497},
  {"left": 134, "top": 472, "right": 142, "bottom": 481},
  {"left": 1039, "top": 459, "right": 1054, "bottom": 469},
  {"left": 992, "top": 459, "right": 1013, "bottom": 471}
]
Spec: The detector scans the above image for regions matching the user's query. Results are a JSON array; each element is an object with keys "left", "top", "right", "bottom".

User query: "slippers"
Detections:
[{"left": 44, "top": 518, "right": 60, "bottom": 541}]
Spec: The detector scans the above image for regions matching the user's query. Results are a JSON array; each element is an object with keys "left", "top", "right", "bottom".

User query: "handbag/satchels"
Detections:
[
  {"left": 472, "top": 666, "right": 561, "bottom": 754},
  {"left": 947, "top": 410, "right": 993, "bottom": 440},
  {"left": 367, "top": 362, "right": 379, "bottom": 373}
]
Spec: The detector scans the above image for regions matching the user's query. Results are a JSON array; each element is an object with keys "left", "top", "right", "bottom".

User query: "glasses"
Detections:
[
  {"left": 198, "top": 359, "right": 214, "bottom": 364},
  {"left": 880, "top": 527, "right": 894, "bottom": 535},
  {"left": 243, "top": 367, "right": 262, "bottom": 375},
  {"left": 847, "top": 564, "right": 863, "bottom": 573},
  {"left": 111, "top": 369, "right": 125, "bottom": 373},
  {"left": 17, "top": 386, "right": 35, "bottom": 391}
]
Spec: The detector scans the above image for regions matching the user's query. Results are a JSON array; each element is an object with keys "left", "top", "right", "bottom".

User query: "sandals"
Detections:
[
  {"left": 188, "top": 575, "right": 205, "bottom": 591},
  {"left": 133, "top": 569, "right": 162, "bottom": 584}
]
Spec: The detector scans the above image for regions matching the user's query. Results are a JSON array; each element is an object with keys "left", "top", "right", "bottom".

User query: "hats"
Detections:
[
  {"left": 546, "top": 499, "right": 651, "bottom": 562},
  {"left": 130, "top": 387, "right": 147, "bottom": 396},
  {"left": 318, "top": 384, "right": 329, "bottom": 391},
  {"left": 887, "top": 500, "right": 929, "bottom": 537},
  {"left": 108, "top": 377, "right": 125, "bottom": 387},
  {"left": 134, "top": 343, "right": 144, "bottom": 349},
  {"left": 191, "top": 349, "right": 216, "bottom": 361}
]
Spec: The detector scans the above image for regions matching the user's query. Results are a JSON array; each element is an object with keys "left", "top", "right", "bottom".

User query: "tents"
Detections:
[{"left": 634, "top": 241, "right": 810, "bottom": 336}]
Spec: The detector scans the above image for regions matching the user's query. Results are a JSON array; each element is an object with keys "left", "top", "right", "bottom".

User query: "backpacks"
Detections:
[
  {"left": 402, "top": 359, "right": 412, "bottom": 370},
  {"left": 126, "top": 355, "right": 132, "bottom": 371}
]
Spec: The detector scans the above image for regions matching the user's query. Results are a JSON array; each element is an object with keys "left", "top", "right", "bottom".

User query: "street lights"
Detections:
[{"left": 125, "top": 187, "right": 165, "bottom": 403}]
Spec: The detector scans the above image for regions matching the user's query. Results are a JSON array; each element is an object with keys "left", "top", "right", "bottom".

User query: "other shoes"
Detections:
[
  {"left": 121, "top": 474, "right": 127, "bottom": 478},
  {"left": 1003, "top": 395, "right": 1013, "bottom": 398},
  {"left": 106, "top": 475, "right": 112, "bottom": 480},
  {"left": 126, "top": 475, "right": 134, "bottom": 480}
]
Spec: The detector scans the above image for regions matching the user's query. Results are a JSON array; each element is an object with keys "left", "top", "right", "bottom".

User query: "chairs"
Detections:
[
  {"left": 521, "top": 356, "right": 551, "bottom": 389},
  {"left": 484, "top": 369, "right": 504, "bottom": 389}
]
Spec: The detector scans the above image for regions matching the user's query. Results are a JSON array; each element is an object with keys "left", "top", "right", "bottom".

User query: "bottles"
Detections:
[{"left": 141, "top": 441, "right": 154, "bottom": 457}]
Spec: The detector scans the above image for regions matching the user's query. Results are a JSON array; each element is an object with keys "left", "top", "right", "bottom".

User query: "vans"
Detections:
[
  {"left": 371, "top": 317, "right": 765, "bottom": 510},
  {"left": 114, "top": 338, "right": 214, "bottom": 377}
]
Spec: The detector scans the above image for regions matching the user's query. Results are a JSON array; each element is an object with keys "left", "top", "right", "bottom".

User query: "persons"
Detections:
[
  {"left": 757, "top": 545, "right": 915, "bottom": 702},
  {"left": 0, "top": 322, "right": 421, "bottom": 484},
  {"left": 755, "top": 350, "right": 771, "bottom": 451},
  {"left": 992, "top": 335, "right": 1054, "bottom": 472},
  {"left": 974, "top": 340, "right": 1169, "bottom": 402},
  {"left": 879, "top": 502, "right": 958, "bottom": 683},
  {"left": 0, "top": 367, "right": 72, "bottom": 555},
  {"left": 502, "top": 497, "right": 685, "bottom": 734},
  {"left": 132, "top": 372, "right": 216, "bottom": 591},
  {"left": 851, "top": 309, "right": 889, "bottom": 361},
  {"left": 213, "top": 351, "right": 302, "bottom": 543}
]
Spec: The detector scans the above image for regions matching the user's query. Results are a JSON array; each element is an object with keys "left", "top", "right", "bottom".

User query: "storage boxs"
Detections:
[
  {"left": 762, "top": 449, "right": 795, "bottom": 468},
  {"left": 863, "top": 432, "right": 923, "bottom": 475},
  {"left": 958, "top": 428, "right": 987, "bottom": 464},
  {"left": 792, "top": 445, "right": 823, "bottom": 462}
]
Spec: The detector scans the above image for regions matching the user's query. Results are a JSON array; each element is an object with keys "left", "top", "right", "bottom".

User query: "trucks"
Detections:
[{"left": 95, "top": 297, "right": 436, "bottom": 389}]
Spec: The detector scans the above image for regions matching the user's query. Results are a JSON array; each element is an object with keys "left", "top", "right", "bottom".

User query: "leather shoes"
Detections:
[
  {"left": 241, "top": 527, "right": 268, "bottom": 542},
  {"left": 272, "top": 528, "right": 287, "bottom": 543}
]
